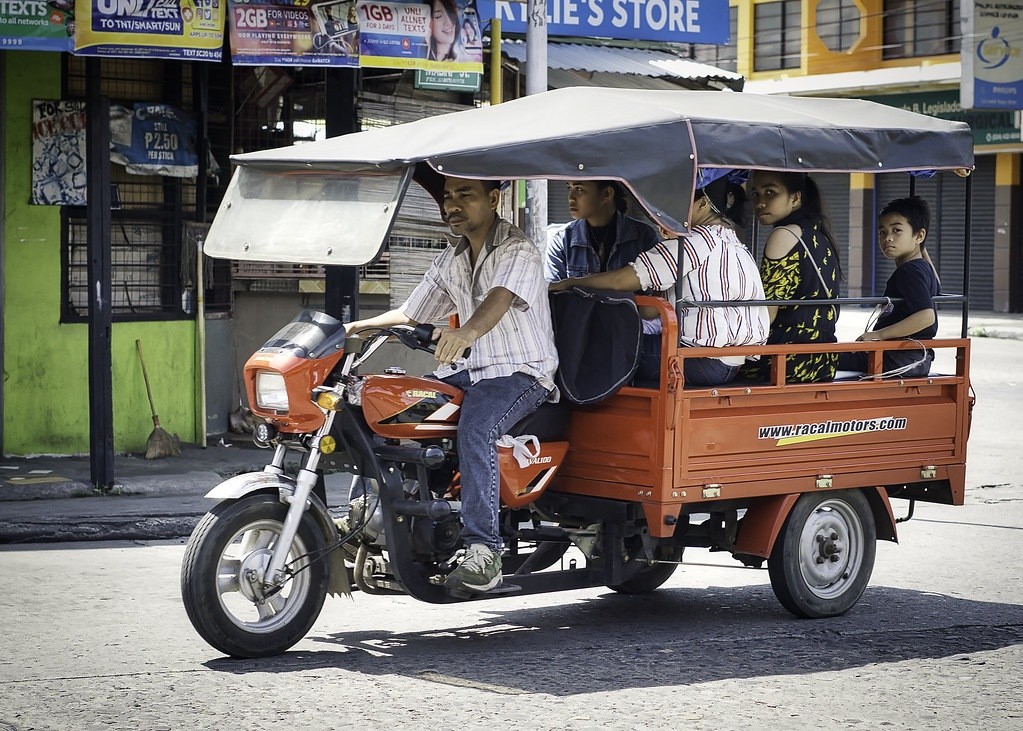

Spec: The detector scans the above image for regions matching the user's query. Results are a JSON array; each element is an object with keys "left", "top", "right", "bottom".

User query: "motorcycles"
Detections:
[{"left": 176, "top": 85, "right": 980, "bottom": 660}]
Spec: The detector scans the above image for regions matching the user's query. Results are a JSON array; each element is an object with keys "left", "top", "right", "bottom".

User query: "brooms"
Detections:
[
  {"left": 226, "top": 308, "right": 265, "bottom": 434},
  {"left": 132, "top": 337, "right": 186, "bottom": 460}
]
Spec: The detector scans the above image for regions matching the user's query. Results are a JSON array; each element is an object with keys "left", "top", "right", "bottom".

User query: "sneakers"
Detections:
[
  {"left": 330, "top": 515, "right": 360, "bottom": 548},
  {"left": 445, "top": 548, "right": 505, "bottom": 593}
]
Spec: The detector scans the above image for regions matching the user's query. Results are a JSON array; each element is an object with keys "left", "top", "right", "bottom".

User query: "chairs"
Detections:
[{"left": 474, "top": 281, "right": 609, "bottom": 441}]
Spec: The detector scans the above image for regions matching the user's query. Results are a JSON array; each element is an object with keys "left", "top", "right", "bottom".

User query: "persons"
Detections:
[
  {"left": 422, "top": 0, "right": 456, "bottom": 62},
  {"left": 462, "top": 21, "right": 477, "bottom": 43},
  {"left": 324, "top": 4, "right": 358, "bottom": 31},
  {"left": 338, "top": 174, "right": 560, "bottom": 599},
  {"left": 838, "top": 197, "right": 940, "bottom": 377},
  {"left": 548, "top": 176, "right": 766, "bottom": 389},
  {"left": 740, "top": 169, "right": 842, "bottom": 384},
  {"left": 544, "top": 181, "right": 661, "bottom": 321}
]
